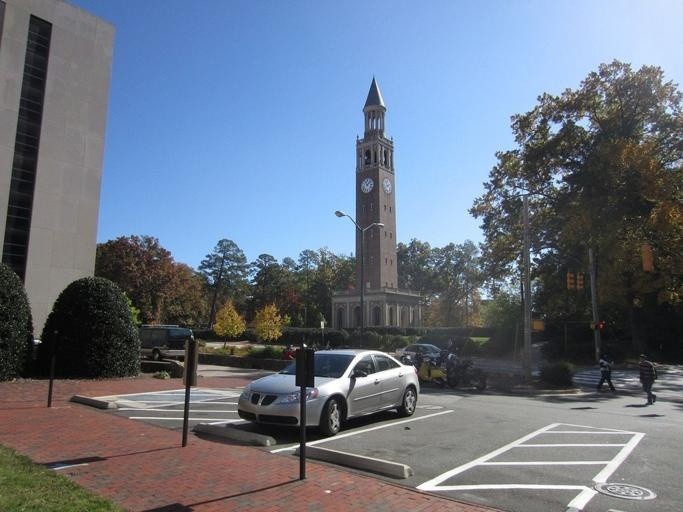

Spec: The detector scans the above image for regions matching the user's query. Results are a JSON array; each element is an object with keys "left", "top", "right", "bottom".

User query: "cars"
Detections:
[
  {"left": 237, "top": 349, "right": 419, "bottom": 437},
  {"left": 395, "top": 344, "right": 456, "bottom": 364}
]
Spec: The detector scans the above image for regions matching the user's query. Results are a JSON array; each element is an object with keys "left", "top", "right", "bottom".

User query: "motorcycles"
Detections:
[{"left": 418, "top": 348, "right": 485, "bottom": 391}]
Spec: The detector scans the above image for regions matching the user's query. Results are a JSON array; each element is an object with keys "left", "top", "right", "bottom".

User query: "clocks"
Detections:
[
  {"left": 360, "top": 177, "right": 374, "bottom": 194},
  {"left": 381, "top": 177, "right": 392, "bottom": 194}
]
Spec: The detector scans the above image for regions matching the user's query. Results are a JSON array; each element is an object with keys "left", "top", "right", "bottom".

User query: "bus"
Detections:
[{"left": 138, "top": 324, "right": 194, "bottom": 361}]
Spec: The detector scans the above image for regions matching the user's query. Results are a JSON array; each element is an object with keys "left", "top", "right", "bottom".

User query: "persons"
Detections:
[
  {"left": 639, "top": 353, "right": 656, "bottom": 405},
  {"left": 284, "top": 341, "right": 332, "bottom": 360},
  {"left": 440, "top": 336, "right": 459, "bottom": 362},
  {"left": 596, "top": 351, "right": 616, "bottom": 392}
]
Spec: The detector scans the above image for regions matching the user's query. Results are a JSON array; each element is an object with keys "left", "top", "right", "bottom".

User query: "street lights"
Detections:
[{"left": 334, "top": 209, "right": 384, "bottom": 349}]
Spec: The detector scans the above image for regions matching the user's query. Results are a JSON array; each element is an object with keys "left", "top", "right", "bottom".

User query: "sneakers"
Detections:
[{"left": 646, "top": 395, "right": 656, "bottom": 405}]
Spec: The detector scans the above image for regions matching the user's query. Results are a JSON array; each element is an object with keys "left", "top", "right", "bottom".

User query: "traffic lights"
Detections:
[
  {"left": 639, "top": 245, "right": 653, "bottom": 273},
  {"left": 597, "top": 322, "right": 605, "bottom": 330},
  {"left": 576, "top": 272, "right": 582, "bottom": 289},
  {"left": 566, "top": 272, "right": 574, "bottom": 289}
]
